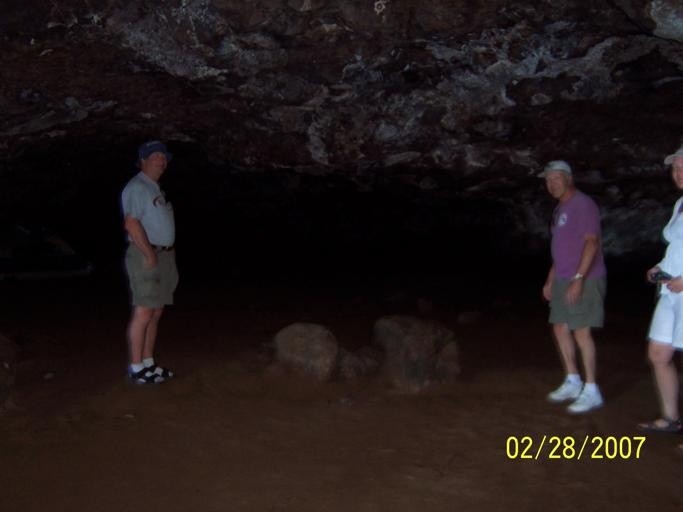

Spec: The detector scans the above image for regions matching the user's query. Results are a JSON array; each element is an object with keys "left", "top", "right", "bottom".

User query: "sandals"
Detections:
[
  {"left": 635, "top": 416, "right": 682, "bottom": 434},
  {"left": 129, "top": 362, "right": 178, "bottom": 384}
]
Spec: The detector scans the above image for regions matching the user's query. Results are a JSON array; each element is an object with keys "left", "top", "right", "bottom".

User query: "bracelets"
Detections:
[{"left": 571, "top": 273, "right": 583, "bottom": 280}]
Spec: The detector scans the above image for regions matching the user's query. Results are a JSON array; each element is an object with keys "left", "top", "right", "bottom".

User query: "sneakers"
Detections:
[{"left": 550, "top": 378, "right": 604, "bottom": 414}]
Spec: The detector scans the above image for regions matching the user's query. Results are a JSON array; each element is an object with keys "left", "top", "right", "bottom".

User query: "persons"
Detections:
[
  {"left": 534, "top": 158, "right": 610, "bottom": 415},
  {"left": 633, "top": 146, "right": 683, "bottom": 454},
  {"left": 119, "top": 139, "right": 179, "bottom": 386}
]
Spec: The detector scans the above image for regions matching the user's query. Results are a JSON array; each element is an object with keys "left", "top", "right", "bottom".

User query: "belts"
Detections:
[{"left": 150, "top": 244, "right": 175, "bottom": 252}]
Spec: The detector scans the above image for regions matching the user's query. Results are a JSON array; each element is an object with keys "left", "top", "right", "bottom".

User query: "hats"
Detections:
[
  {"left": 138, "top": 140, "right": 173, "bottom": 162},
  {"left": 665, "top": 147, "right": 682, "bottom": 165},
  {"left": 537, "top": 161, "right": 572, "bottom": 178}
]
